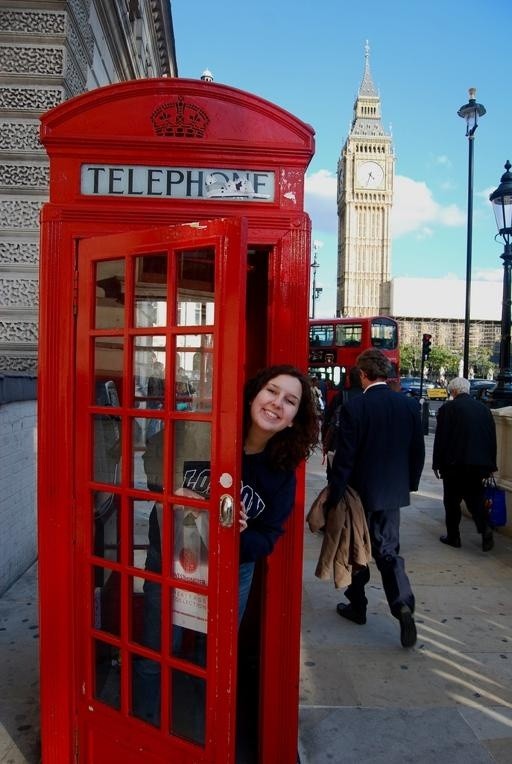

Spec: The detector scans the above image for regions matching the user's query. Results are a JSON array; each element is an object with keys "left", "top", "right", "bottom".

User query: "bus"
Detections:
[{"left": 309, "top": 315, "right": 403, "bottom": 422}]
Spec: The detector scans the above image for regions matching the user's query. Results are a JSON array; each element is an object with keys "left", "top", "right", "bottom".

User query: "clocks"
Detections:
[{"left": 356, "top": 159, "right": 386, "bottom": 192}]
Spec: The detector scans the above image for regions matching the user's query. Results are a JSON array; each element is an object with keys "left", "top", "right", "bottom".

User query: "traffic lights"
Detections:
[{"left": 422, "top": 333, "right": 433, "bottom": 356}]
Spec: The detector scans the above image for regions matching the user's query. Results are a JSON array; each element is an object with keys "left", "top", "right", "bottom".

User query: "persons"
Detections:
[
  {"left": 325, "top": 346, "right": 426, "bottom": 649},
  {"left": 430, "top": 375, "right": 499, "bottom": 553},
  {"left": 307, "top": 363, "right": 365, "bottom": 481},
  {"left": 133, "top": 360, "right": 214, "bottom": 443},
  {"left": 128, "top": 360, "right": 320, "bottom": 747}
]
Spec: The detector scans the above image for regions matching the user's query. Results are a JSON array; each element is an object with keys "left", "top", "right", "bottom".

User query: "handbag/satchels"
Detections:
[{"left": 482, "top": 473, "right": 507, "bottom": 528}]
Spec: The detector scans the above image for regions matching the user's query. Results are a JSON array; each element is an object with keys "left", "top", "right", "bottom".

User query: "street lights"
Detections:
[
  {"left": 310, "top": 236, "right": 323, "bottom": 317},
  {"left": 458, "top": 83, "right": 485, "bottom": 387},
  {"left": 488, "top": 158, "right": 512, "bottom": 410}
]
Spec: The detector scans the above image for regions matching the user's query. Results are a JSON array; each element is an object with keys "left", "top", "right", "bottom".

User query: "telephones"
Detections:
[{"left": 94, "top": 381, "right": 121, "bottom": 472}]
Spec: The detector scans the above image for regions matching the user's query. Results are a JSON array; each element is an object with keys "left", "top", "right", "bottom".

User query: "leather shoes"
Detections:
[
  {"left": 398, "top": 604, "right": 417, "bottom": 649},
  {"left": 440, "top": 536, "right": 461, "bottom": 548},
  {"left": 481, "top": 527, "right": 495, "bottom": 552},
  {"left": 337, "top": 602, "right": 366, "bottom": 624}
]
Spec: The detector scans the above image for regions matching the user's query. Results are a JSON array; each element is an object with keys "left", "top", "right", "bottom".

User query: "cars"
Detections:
[{"left": 398, "top": 375, "right": 498, "bottom": 408}]
